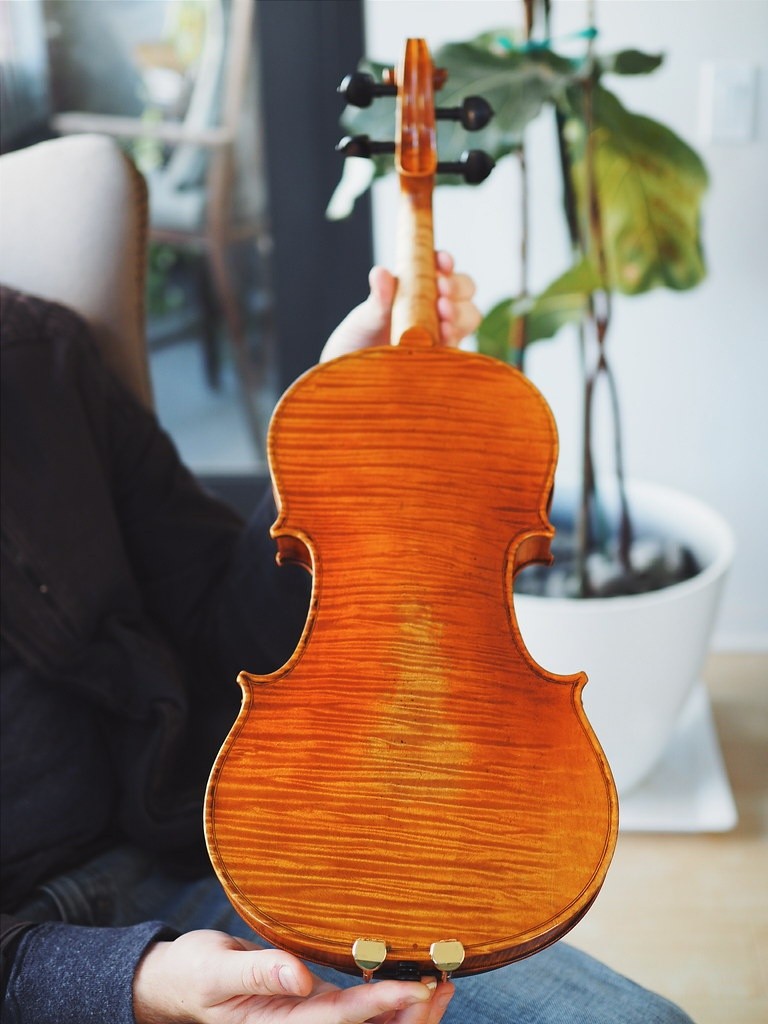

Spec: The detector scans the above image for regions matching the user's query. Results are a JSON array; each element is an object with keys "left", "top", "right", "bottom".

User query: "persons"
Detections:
[{"left": 0, "top": 135, "right": 698, "bottom": 1022}]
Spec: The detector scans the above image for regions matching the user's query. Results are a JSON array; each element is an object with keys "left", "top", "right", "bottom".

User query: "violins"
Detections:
[{"left": 203, "top": 36, "right": 623, "bottom": 982}]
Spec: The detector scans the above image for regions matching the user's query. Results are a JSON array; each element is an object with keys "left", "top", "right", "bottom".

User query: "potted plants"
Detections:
[{"left": 337, "top": 0, "right": 739, "bottom": 795}]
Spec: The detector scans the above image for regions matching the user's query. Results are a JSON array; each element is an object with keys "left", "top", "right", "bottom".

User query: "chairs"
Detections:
[{"left": 0, "top": 132, "right": 155, "bottom": 410}]
